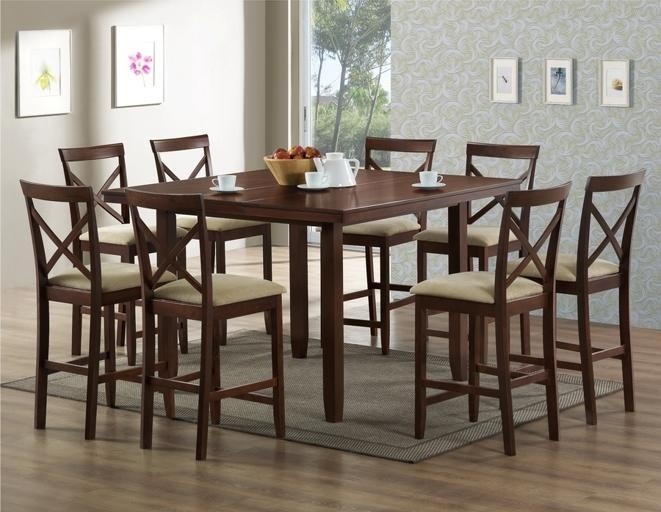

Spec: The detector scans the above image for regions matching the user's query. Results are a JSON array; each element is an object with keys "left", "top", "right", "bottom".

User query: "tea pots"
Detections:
[{"left": 311, "top": 152, "right": 360, "bottom": 189}]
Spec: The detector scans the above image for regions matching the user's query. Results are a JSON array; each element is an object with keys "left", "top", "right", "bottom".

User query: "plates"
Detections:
[
  {"left": 297, "top": 184, "right": 328, "bottom": 191},
  {"left": 209, "top": 186, "right": 244, "bottom": 193},
  {"left": 411, "top": 183, "right": 446, "bottom": 189}
]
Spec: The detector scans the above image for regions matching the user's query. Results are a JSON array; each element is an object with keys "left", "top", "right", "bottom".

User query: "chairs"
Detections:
[
  {"left": 499, "top": 167, "right": 648, "bottom": 426},
  {"left": 121, "top": 186, "right": 287, "bottom": 461},
  {"left": 316, "top": 137, "right": 438, "bottom": 356},
  {"left": 411, "top": 140, "right": 542, "bottom": 358},
  {"left": 148, "top": 134, "right": 272, "bottom": 346},
  {"left": 19, "top": 177, "right": 176, "bottom": 441},
  {"left": 56, "top": 142, "right": 190, "bottom": 356},
  {"left": 414, "top": 181, "right": 573, "bottom": 457}
]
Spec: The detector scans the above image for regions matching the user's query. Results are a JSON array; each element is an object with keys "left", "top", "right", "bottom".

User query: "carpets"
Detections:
[{"left": 2, "top": 328, "right": 627, "bottom": 463}]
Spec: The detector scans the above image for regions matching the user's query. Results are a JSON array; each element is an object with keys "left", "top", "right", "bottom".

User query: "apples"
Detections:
[{"left": 271, "top": 145, "right": 321, "bottom": 159}]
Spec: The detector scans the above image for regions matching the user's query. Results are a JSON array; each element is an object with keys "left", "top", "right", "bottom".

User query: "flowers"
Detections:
[
  {"left": 33, "top": 65, "right": 61, "bottom": 96},
  {"left": 128, "top": 51, "right": 155, "bottom": 89}
]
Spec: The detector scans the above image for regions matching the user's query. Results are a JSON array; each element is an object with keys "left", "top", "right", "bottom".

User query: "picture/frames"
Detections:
[
  {"left": 114, "top": 24, "right": 164, "bottom": 108},
  {"left": 16, "top": 29, "right": 72, "bottom": 117},
  {"left": 599, "top": 59, "right": 632, "bottom": 108},
  {"left": 543, "top": 58, "right": 575, "bottom": 107},
  {"left": 489, "top": 57, "right": 520, "bottom": 105}
]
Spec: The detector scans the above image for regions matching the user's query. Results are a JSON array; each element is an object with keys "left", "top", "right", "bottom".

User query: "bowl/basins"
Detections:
[{"left": 263, "top": 154, "right": 316, "bottom": 186}]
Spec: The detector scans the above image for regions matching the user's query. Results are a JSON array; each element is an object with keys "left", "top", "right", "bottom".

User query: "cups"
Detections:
[
  {"left": 211, "top": 175, "right": 237, "bottom": 189},
  {"left": 304, "top": 171, "right": 329, "bottom": 186},
  {"left": 419, "top": 170, "right": 442, "bottom": 184}
]
